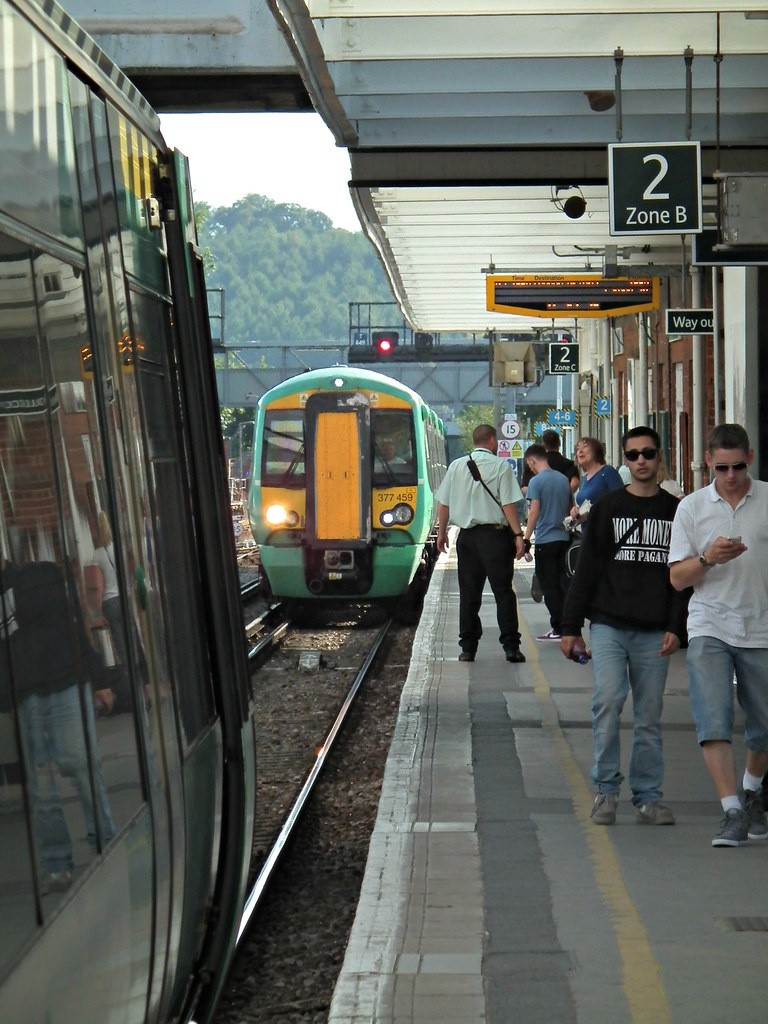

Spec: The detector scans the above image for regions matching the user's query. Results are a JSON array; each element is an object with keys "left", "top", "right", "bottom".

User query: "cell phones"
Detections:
[{"left": 727, "top": 535, "right": 742, "bottom": 544}]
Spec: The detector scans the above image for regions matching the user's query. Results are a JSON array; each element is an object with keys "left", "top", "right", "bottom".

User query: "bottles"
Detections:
[
  {"left": 524, "top": 552, "right": 533, "bottom": 562},
  {"left": 571, "top": 644, "right": 588, "bottom": 664}
]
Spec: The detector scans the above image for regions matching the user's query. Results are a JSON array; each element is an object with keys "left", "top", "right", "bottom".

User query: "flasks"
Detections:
[{"left": 93, "top": 626, "right": 122, "bottom": 672}]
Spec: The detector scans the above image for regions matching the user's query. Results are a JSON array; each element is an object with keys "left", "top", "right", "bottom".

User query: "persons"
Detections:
[
  {"left": 434, "top": 424, "right": 524, "bottom": 662},
  {"left": 1, "top": 513, "right": 150, "bottom": 894},
  {"left": 524, "top": 430, "right": 686, "bottom": 645},
  {"left": 560, "top": 427, "right": 695, "bottom": 822},
  {"left": 668, "top": 424, "right": 768, "bottom": 847}
]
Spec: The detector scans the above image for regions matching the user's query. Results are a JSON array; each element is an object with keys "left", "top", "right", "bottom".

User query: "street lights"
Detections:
[{"left": 239, "top": 421, "right": 256, "bottom": 489}]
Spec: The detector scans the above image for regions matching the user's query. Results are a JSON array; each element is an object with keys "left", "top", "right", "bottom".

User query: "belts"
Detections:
[{"left": 473, "top": 524, "right": 509, "bottom": 534}]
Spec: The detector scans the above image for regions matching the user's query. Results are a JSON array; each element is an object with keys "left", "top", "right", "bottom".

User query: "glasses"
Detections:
[
  {"left": 624, "top": 448, "right": 657, "bottom": 462},
  {"left": 712, "top": 462, "right": 748, "bottom": 472}
]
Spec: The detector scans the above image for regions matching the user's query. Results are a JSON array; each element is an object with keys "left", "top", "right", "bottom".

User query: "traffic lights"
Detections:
[{"left": 371, "top": 331, "right": 401, "bottom": 358}]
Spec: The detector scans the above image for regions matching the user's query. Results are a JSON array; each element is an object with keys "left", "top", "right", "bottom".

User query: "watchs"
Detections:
[
  {"left": 515, "top": 531, "right": 524, "bottom": 537},
  {"left": 700, "top": 550, "right": 717, "bottom": 568}
]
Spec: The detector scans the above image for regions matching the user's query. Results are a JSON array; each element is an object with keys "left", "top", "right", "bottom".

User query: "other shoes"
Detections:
[{"left": 531, "top": 574, "right": 543, "bottom": 603}]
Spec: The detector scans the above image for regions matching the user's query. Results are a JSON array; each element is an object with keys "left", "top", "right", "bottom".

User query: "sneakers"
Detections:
[
  {"left": 590, "top": 790, "right": 618, "bottom": 825},
  {"left": 535, "top": 629, "right": 562, "bottom": 643},
  {"left": 736, "top": 778, "right": 768, "bottom": 839},
  {"left": 636, "top": 800, "right": 675, "bottom": 825},
  {"left": 711, "top": 808, "right": 749, "bottom": 846}
]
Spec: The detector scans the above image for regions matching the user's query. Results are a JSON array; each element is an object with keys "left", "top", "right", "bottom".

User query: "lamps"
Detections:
[
  {"left": 556, "top": 186, "right": 587, "bottom": 219},
  {"left": 586, "top": 90, "right": 615, "bottom": 111}
]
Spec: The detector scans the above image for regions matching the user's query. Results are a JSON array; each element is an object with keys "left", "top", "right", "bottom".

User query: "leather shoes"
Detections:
[
  {"left": 506, "top": 649, "right": 525, "bottom": 663},
  {"left": 459, "top": 652, "right": 475, "bottom": 662}
]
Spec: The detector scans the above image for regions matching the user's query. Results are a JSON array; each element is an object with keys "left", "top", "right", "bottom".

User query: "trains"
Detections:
[
  {"left": 2, "top": 0, "right": 257, "bottom": 1024},
  {"left": 247, "top": 363, "right": 462, "bottom": 617}
]
